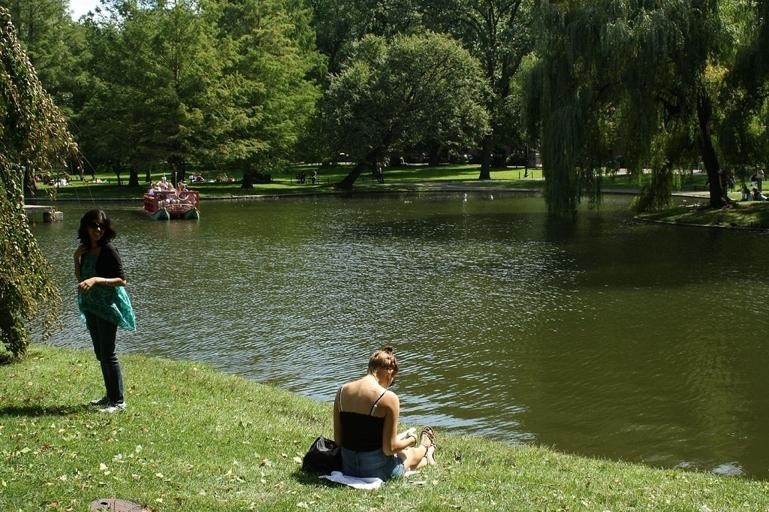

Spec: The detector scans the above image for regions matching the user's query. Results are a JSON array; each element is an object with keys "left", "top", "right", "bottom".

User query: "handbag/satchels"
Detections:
[{"left": 301, "top": 435, "right": 342, "bottom": 477}]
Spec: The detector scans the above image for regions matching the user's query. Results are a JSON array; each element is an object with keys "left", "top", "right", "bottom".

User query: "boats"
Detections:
[{"left": 143, "top": 189, "right": 200, "bottom": 222}]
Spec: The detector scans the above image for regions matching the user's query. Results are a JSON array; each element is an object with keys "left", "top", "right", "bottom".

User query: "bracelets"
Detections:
[{"left": 407, "top": 435, "right": 417, "bottom": 447}]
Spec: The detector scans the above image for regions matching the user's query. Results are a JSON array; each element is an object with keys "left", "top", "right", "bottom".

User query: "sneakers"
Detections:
[{"left": 90, "top": 396, "right": 127, "bottom": 414}]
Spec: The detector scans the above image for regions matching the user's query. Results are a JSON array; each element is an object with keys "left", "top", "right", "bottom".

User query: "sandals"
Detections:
[{"left": 420, "top": 425, "right": 437, "bottom": 465}]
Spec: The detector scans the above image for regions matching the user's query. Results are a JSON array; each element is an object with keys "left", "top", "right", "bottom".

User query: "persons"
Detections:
[
  {"left": 300, "top": 170, "right": 306, "bottom": 182},
  {"left": 740, "top": 185, "right": 751, "bottom": 201},
  {"left": 311, "top": 169, "right": 315, "bottom": 183},
  {"left": 375, "top": 167, "right": 385, "bottom": 182},
  {"left": 34, "top": 172, "right": 109, "bottom": 187},
  {"left": 147, "top": 174, "right": 204, "bottom": 211},
  {"left": 752, "top": 187, "right": 762, "bottom": 201},
  {"left": 754, "top": 165, "right": 764, "bottom": 191},
  {"left": 70, "top": 207, "right": 133, "bottom": 415},
  {"left": 332, "top": 344, "right": 439, "bottom": 485}
]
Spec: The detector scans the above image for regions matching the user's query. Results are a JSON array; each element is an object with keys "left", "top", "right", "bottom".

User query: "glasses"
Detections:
[
  {"left": 90, "top": 223, "right": 105, "bottom": 230},
  {"left": 389, "top": 379, "right": 396, "bottom": 387}
]
